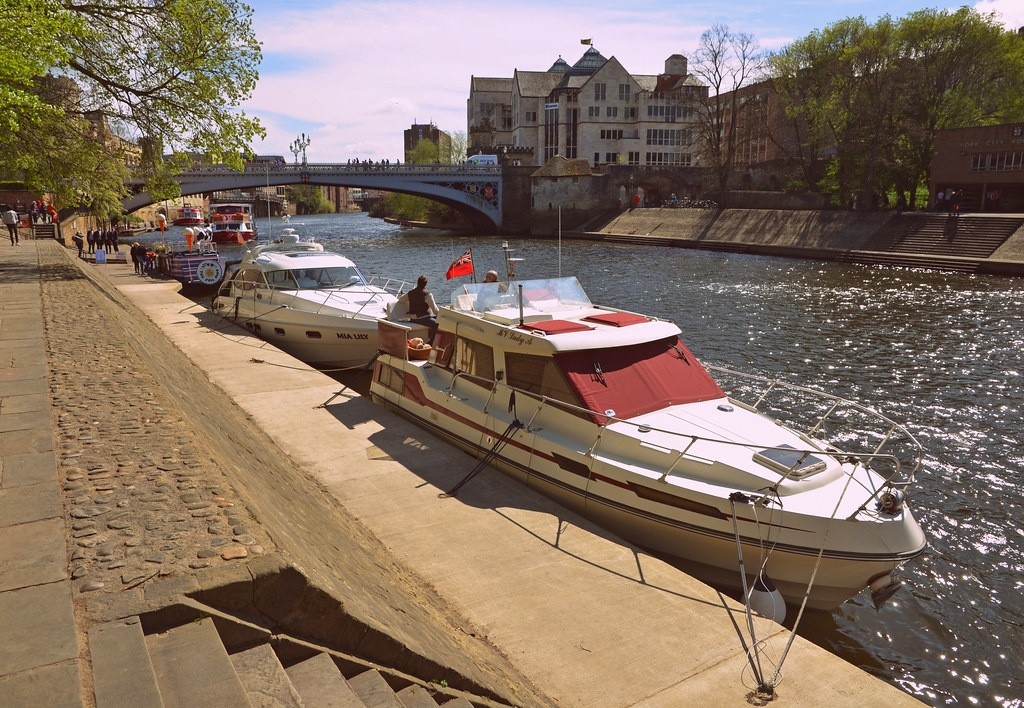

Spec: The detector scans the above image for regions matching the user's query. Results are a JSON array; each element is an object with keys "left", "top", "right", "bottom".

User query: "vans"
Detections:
[{"left": 464, "top": 155, "right": 498, "bottom": 166}]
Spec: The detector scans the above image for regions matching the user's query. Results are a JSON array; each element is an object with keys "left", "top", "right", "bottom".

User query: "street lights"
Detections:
[
  {"left": 297, "top": 133, "right": 311, "bottom": 163},
  {"left": 290, "top": 135, "right": 301, "bottom": 165}
]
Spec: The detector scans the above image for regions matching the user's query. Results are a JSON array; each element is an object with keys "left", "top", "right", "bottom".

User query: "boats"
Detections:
[
  {"left": 203, "top": 203, "right": 260, "bottom": 245},
  {"left": 146, "top": 213, "right": 226, "bottom": 290},
  {"left": 210, "top": 224, "right": 415, "bottom": 379},
  {"left": 368, "top": 204, "right": 931, "bottom": 625},
  {"left": 176, "top": 204, "right": 206, "bottom": 226}
]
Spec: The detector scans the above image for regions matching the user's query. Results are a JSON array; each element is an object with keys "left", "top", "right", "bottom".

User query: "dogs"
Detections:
[{"left": 408, "top": 337, "right": 431, "bottom": 350}]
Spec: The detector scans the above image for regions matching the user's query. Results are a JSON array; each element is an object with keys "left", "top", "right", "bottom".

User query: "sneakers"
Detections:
[{"left": 11, "top": 242, "right": 22, "bottom": 246}]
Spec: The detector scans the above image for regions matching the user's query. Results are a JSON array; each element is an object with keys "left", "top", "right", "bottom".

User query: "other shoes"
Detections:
[
  {"left": 106, "top": 251, "right": 109, "bottom": 253},
  {"left": 34, "top": 222, "right": 38, "bottom": 225},
  {"left": 136, "top": 271, "right": 145, "bottom": 276},
  {"left": 42, "top": 222, "right": 45, "bottom": 223},
  {"left": 78, "top": 253, "right": 81, "bottom": 257},
  {"left": 88, "top": 251, "right": 93, "bottom": 254},
  {"left": 48, "top": 221, "right": 51, "bottom": 224}
]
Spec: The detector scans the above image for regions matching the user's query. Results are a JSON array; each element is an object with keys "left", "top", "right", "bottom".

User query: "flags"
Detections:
[{"left": 446, "top": 251, "right": 474, "bottom": 280}]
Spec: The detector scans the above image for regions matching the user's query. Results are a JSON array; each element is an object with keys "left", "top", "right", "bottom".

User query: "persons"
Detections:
[
  {"left": 670, "top": 193, "right": 677, "bottom": 204},
  {"left": 399, "top": 276, "right": 439, "bottom": 346},
  {"left": 273, "top": 157, "right": 286, "bottom": 176},
  {"left": 948, "top": 189, "right": 963, "bottom": 217},
  {"left": 31, "top": 200, "right": 56, "bottom": 224},
  {"left": 477, "top": 270, "right": 507, "bottom": 311},
  {"left": 75, "top": 231, "right": 84, "bottom": 258},
  {"left": 4, "top": 205, "right": 21, "bottom": 246},
  {"left": 87, "top": 226, "right": 119, "bottom": 255},
  {"left": 130, "top": 242, "right": 152, "bottom": 277},
  {"left": 937, "top": 188, "right": 944, "bottom": 211},
  {"left": 634, "top": 192, "right": 640, "bottom": 208},
  {"left": 348, "top": 158, "right": 400, "bottom": 172},
  {"left": 643, "top": 193, "right": 656, "bottom": 208}
]
